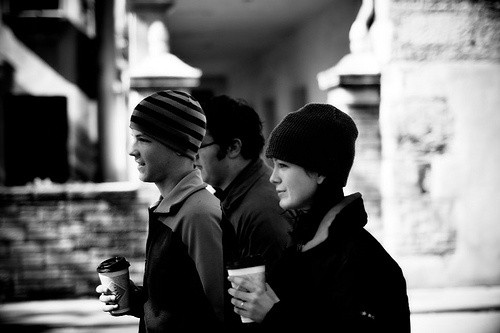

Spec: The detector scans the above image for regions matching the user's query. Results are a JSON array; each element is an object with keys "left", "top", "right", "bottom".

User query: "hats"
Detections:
[
  {"left": 130, "top": 90, "right": 206, "bottom": 160},
  {"left": 265, "top": 104, "right": 358, "bottom": 188}
]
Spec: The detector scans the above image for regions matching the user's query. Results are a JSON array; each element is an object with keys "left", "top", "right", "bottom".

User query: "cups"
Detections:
[
  {"left": 226, "top": 260, "right": 265, "bottom": 323},
  {"left": 96, "top": 255, "right": 130, "bottom": 314}
]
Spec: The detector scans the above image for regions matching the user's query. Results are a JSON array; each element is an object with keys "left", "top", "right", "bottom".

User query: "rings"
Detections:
[{"left": 241, "top": 302, "right": 244, "bottom": 308}]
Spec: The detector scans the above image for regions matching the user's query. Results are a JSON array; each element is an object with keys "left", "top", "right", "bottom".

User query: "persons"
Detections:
[
  {"left": 94, "top": 88, "right": 224, "bottom": 333},
  {"left": 196, "top": 95, "right": 292, "bottom": 255},
  {"left": 227, "top": 103, "right": 411, "bottom": 333}
]
